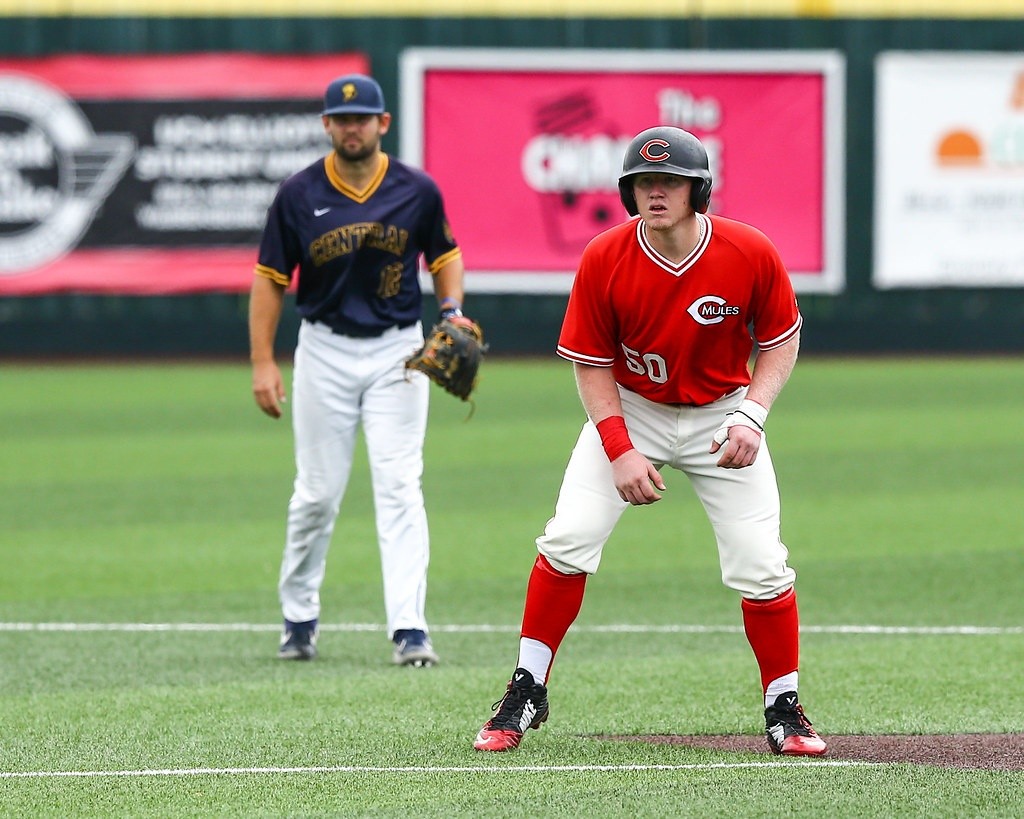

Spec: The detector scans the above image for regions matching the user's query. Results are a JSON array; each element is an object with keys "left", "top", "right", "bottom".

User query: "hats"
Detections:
[{"left": 321, "top": 74, "right": 385, "bottom": 117}]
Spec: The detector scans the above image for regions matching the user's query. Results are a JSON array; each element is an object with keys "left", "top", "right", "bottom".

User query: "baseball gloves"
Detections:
[{"left": 401, "top": 306, "right": 490, "bottom": 423}]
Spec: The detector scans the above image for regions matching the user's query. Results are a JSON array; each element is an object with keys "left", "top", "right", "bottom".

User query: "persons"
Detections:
[
  {"left": 474, "top": 126, "right": 829, "bottom": 757},
  {"left": 248, "top": 75, "right": 465, "bottom": 665}
]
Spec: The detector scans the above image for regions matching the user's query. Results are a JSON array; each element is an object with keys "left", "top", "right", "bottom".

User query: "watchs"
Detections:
[{"left": 440, "top": 307, "right": 462, "bottom": 318}]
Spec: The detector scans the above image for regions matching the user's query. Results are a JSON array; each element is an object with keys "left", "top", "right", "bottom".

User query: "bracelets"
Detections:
[{"left": 595, "top": 416, "right": 634, "bottom": 462}]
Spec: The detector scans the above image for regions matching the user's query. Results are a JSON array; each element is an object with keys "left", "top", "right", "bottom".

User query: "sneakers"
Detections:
[
  {"left": 765, "top": 690, "right": 830, "bottom": 756},
  {"left": 393, "top": 628, "right": 440, "bottom": 670},
  {"left": 278, "top": 619, "right": 319, "bottom": 659},
  {"left": 473, "top": 668, "right": 549, "bottom": 751}
]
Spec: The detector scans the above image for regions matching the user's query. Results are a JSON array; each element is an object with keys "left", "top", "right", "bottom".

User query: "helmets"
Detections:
[{"left": 618, "top": 126, "right": 713, "bottom": 217}]
